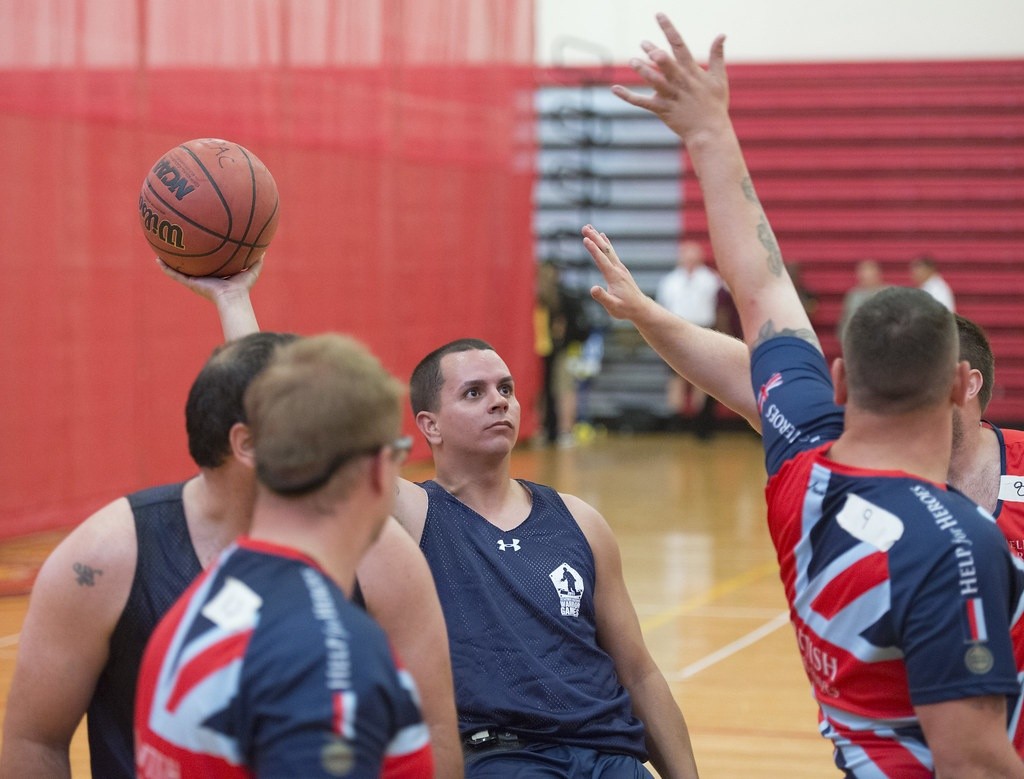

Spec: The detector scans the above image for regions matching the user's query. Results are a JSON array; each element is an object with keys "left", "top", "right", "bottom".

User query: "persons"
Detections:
[
  {"left": 0, "top": 251, "right": 703, "bottom": 779},
  {"left": 582, "top": 9, "right": 1024, "bottom": 779}
]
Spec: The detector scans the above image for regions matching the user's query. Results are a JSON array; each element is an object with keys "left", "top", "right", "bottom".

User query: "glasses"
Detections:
[{"left": 372, "top": 435, "right": 413, "bottom": 467}]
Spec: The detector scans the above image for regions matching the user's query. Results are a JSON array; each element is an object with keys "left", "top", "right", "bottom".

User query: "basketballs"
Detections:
[{"left": 136, "top": 136, "right": 280, "bottom": 278}]
